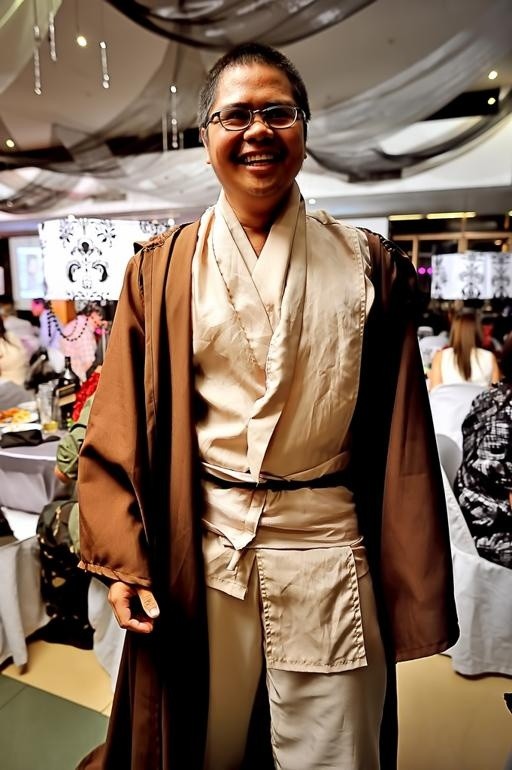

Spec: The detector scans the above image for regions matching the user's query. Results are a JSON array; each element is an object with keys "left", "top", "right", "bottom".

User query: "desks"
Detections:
[{"left": 0, "top": 434, "right": 66, "bottom": 517}]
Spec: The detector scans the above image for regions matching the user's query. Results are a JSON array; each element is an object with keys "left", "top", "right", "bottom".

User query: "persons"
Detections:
[
  {"left": 76, "top": 37, "right": 461, "bottom": 769},
  {"left": 22, "top": 297, "right": 60, "bottom": 350},
  {"left": 0, "top": 315, "right": 29, "bottom": 383},
  {"left": 454, "top": 328, "right": 510, "bottom": 570},
  {"left": 430, "top": 312, "right": 500, "bottom": 394},
  {"left": 35, "top": 390, "right": 97, "bottom": 649},
  {"left": 59, "top": 305, "right": 105, "bottom": 375},
  {"left": 1, "top": 295, "right": 31, "bottom": 337}
]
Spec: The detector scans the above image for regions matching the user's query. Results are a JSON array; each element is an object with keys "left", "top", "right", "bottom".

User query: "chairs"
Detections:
[
  {"left": 0, "top": 500, "right": 50, "bottom": 667},
  {"left": 434, "top": 436, "right": 512, "bottom": 676},
  {"left": 429, "top": 382, "right": 490, "bottom": 447}
]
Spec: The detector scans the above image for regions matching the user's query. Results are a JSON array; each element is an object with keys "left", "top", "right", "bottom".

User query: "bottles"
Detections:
[{"left": 59, "top": 357, "right": 80, "bottom": 394}]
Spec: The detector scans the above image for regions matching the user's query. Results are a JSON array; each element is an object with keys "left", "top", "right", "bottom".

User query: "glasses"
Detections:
[{"left": 206, "top": 105, "right": 305, "bottom": 131}]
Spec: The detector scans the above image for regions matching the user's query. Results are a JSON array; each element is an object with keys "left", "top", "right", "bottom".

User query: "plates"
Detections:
[
  {"left": 0, "top": 412, "right": 39, "bottom": 428},
  {"left": 2, "top": 423, "right": 42, "bottom": 433}
]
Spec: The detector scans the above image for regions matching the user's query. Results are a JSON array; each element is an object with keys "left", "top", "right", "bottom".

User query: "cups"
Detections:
[{"left": 37, "top": 393, "right": 60, "bottom": 434}]
